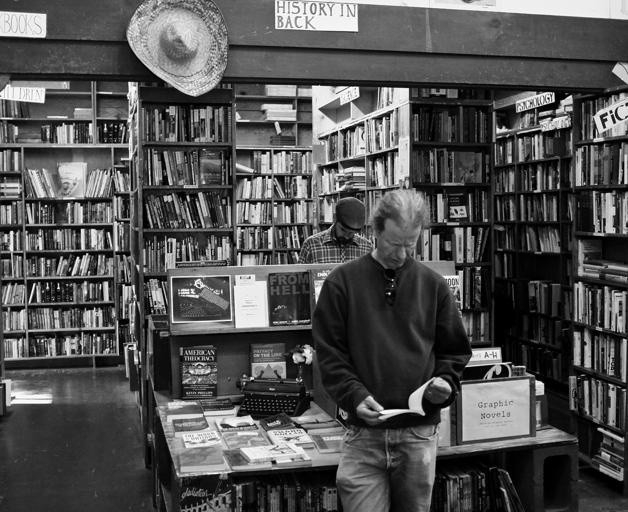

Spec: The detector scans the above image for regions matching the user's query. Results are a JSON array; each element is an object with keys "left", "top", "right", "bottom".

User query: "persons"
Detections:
[{"left": 310, "top": 187, "right": 474, "bottom": 512}]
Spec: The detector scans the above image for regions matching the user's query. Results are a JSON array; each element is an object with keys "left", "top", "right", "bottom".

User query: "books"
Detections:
[
  {"left": 495, "top": 86, "right": 627, "bottom": 482},
  {"left": 376, "top": 375, "right": 436, "bottom": 422},
  {"left": 165, "top": 358, "right": 355, "bottom": 512},
  {"left": 139, "top": 82, "right": 494, "bottom": 359},
  {"left": 426, "top": 461, "right": 524, "bottom": 512},
  {"left": 0, "top": 80, "right": 140, "bottom": 358}
]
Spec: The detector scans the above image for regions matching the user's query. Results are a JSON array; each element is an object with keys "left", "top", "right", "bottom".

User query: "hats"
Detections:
[
  {"left": 126, "top": 0, "right": 230, "bottom": 97},
  {"left": 337, "top": 197, "right": 365, "bottom": 230}
]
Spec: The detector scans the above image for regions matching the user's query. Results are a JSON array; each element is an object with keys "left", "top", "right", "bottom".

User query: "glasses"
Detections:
[{"left": 382, "top": 269, "right": 397, "bottom": 307}]
